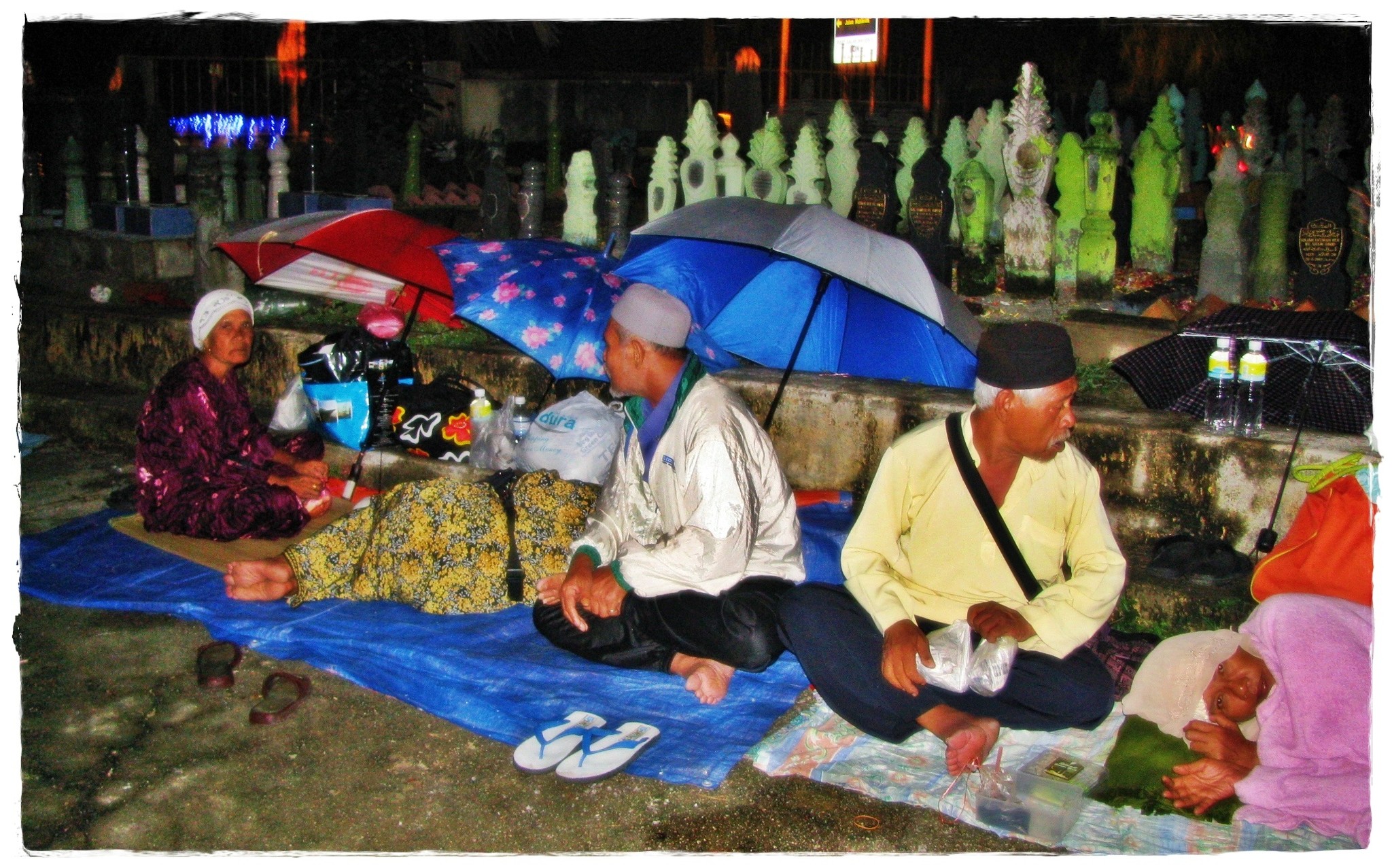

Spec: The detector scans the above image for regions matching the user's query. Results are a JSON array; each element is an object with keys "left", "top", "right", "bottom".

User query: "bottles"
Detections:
[
  {"left": 1233, "top": 341, "right": 1267, "bottom": 437},
  {"left": 469, "top": 388, "right": 492, "bottom": 445},
  {"left": 1204, "top": 338, "right": 1235, "bottom": 432},
  {"left": 513, "top": 396, "right": 531, "bottom": 445}
]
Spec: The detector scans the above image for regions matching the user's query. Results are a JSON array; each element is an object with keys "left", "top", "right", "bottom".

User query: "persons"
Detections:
[
  {"left": 225, "top": 466, "right": 602, "bottom": 609},
  {"left": 774, "top": 322, "right": 1126, "bottom": 779},
  {"left": 136, "top": 288, "right": 328, "bottom": 542},
  {"left": 533, "top": 284, "right": 807, "bottom": 704},
  {"left": 1121, "top": 593, "right": 1374, "bottom": 855}
]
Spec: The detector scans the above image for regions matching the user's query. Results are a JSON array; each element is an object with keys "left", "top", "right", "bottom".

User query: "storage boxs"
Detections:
[
  {"left": 92, "top": 204, "right": 195, "bottom": 236},
  {"left": 975, "top": 748, "right": 1105, "bottom": 843},
  {"left": 278, "top": 192, "right": 394, "bottom": 217}
]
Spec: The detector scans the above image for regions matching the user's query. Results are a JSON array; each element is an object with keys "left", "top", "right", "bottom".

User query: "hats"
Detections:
[
  {"left": 975, "top": 321, "right": 1077, "bottom": 389},
  {"left": 192, "top": 289, "right": 256, "bottom": 350},
  {"left": 611, "top": 282, "right": 692, "bottom": 348}
]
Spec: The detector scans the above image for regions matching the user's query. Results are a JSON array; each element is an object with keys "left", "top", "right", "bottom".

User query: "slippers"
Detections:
[
  {"left": 555, "top": 721, "right": 661, "bottom": 783},
  {"left": 513, "top": 711, "right": 606, "bottom": 775},
  {"left": 249, "top": 673, "right": 313, "bottom": 726},
  {"left": 195, "top": 640, "right": 240, "bottom": 690}
]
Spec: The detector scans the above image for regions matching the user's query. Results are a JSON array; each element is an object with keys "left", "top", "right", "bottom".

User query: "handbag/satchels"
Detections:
[
  {"left": 512, "top": 391, "right": 626, "bottom": 485},
  {"left": 1250, "top": 454, "right": 1382, "bottom": 606},
  {"left": 295, "top": 324, "right": 503, "bottom": 464}
]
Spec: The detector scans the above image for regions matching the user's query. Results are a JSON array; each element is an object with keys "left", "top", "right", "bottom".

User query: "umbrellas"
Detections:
[
  {"left": 427, "top": 232, "right": 620, "bottom": 425},
  {"left": 211, "top": 207, "right": 472, "bottom": 502},
  {"left": 1110, "top": 305, "right": 1374, "bottom": 568},
  {"left": 604, "top": 195, "right": 988, "bottom": 432}
]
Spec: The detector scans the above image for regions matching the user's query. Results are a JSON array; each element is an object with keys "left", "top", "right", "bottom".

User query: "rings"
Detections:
[{"left": 313, "top": 484, "right": 316, "bottom": 489}]
